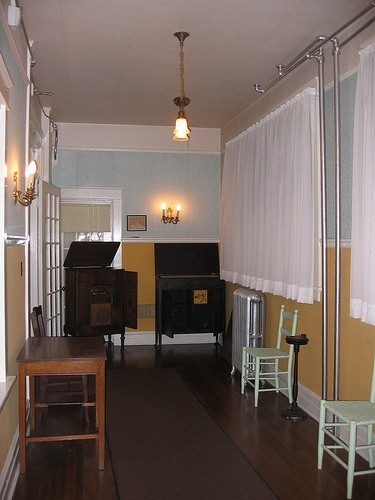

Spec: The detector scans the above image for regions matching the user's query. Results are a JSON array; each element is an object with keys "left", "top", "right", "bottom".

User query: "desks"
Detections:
[{"left": 17, "top": 336, "right": 106, "bottom": 475}]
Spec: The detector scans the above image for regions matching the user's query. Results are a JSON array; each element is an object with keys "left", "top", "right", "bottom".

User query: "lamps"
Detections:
[
  {"left": 160, "top": 202, "right": 181, "bottom": 224},
  {"left": 13, "top": 160, "right": 38, "bottom": 207},
  {"left": 171, "top": 31, "right": 190, "bottom": 141}
]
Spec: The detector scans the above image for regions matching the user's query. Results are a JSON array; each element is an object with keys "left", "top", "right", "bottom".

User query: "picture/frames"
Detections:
[{"left": 127, "top": 215, "right": 147, "bottom": 231}]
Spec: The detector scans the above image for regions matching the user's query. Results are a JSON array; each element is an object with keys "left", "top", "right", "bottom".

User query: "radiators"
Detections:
[{"left": 230, "top": 288, "right": 266, "bottom": 386}]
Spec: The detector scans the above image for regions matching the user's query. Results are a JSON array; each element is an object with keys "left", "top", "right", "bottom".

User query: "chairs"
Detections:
[
  {"left": 30, "top": 305, "right": 92, "bottom": 425},
  {"left": 241, "top": 304, "right": 299, "bottom": 408},
  {"left": 318, "top": 351, "right": 375, "bottom": 500}
]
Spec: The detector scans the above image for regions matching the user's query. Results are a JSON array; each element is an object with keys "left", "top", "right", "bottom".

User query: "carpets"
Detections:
[{"left": 105, "top": 366, "right": 282, "bottom": 500}]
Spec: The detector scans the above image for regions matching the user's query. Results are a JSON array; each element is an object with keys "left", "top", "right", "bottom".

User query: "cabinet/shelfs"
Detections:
[{"left": 63, "top": 241, "right": 227, "bottom": 359}]
